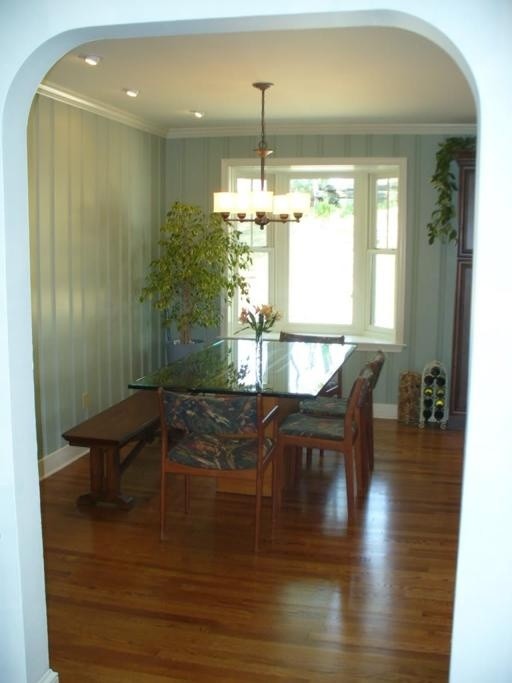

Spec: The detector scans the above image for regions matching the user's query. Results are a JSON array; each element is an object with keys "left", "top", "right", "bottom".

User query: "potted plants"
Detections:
[
  {"left": 426, "top": 135, "right": 476, "bottom": 247},
  {"left": 138, "top": 202, "right": 254, "bottom": 366}
]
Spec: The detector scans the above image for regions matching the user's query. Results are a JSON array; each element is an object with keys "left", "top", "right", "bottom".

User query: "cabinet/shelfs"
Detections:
[{"left": 446, "top": 160, "right": 476, "bottom": 434}]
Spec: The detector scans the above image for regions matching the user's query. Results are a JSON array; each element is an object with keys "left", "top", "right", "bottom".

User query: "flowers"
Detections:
[{"left": 236, "top": 304, "right": 284, "bottom": 342}]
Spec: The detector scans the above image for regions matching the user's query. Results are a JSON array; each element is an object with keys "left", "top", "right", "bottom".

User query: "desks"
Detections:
[{"left": 128, "top": 339, "right": 358, "bottom": 498}]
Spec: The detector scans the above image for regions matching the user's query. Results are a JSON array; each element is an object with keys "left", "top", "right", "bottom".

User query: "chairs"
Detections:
[
  {"left": 159, "top": 387, "right": 280, "bottom": 553},
  {"left": 279, "top": 331, "right": 346, "bottom": 465},
  {"left": 277, "top": 366, "right": 377, "bottom": 518},
  {"left": 298, "top": 349, "right": 385, "bottom": 473}
]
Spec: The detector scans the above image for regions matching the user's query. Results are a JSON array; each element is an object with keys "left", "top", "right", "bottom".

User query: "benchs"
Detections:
[{"left": 62, "top": 390, "right": 161, "bottom": 511}]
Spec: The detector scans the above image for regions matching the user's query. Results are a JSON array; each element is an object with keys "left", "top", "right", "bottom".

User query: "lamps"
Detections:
[{"left": 212, "top": 82, "right": 310, "bottom": 226}]
